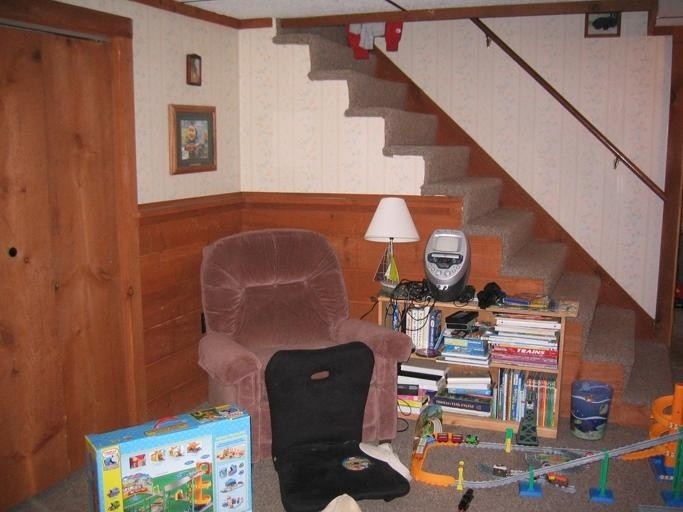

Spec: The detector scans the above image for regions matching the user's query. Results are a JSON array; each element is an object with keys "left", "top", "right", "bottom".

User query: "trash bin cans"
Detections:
[{"left": 571, "top": 380, "right": 612, "bottom": 441}]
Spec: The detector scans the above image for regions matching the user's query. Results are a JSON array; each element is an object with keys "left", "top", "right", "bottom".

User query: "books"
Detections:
[{"left": 390, "top": 307, "right": 562, "bottom": 431}]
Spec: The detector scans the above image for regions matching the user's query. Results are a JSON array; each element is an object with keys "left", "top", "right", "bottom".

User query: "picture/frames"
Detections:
[
  {"left": 583, "top": 12, "right": 622, "bottom": 38},
  {"left": 167, "top": 103, "right": 217, "bottom": 175},
  {"left": 185, "top": 52, "right": 201, "bottom": 86}
]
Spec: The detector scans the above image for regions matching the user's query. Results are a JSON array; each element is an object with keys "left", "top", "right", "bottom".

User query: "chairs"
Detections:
[{"left": 186, "top": 227, "right": 415, "bottom": 463}]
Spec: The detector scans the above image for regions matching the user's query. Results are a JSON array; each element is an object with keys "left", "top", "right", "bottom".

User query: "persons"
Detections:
[{"left": 183, "top": 124, "right": 203, "bottom": 161}]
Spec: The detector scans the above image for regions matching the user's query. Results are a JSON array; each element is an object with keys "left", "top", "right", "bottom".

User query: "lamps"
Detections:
[{"left": 362, "top": 196, "right": 421, "bottom": 288}]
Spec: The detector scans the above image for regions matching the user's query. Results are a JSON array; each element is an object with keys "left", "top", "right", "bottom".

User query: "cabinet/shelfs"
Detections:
[{"left": 376, "top": 283, "right": 580, "bottom": 440}]
